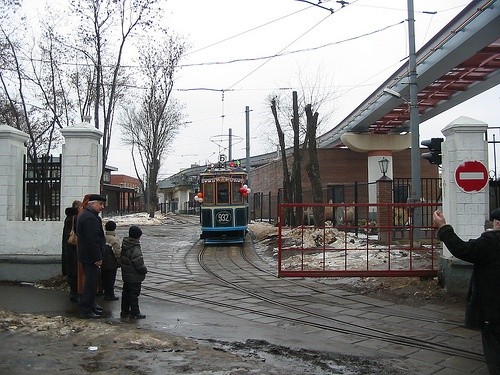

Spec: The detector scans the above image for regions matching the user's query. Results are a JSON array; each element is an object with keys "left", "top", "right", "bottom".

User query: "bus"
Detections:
[{"left": 197, "top": 153, "right": 251, "bottom": 245}]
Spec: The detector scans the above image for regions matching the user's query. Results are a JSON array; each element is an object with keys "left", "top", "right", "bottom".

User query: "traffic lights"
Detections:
[{"left": 420, "top": 137, "right": 444, "bottom": 166}]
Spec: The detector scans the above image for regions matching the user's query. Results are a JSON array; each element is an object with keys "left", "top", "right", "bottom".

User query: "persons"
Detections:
[
  {"left": 61, "top": 200, "right": 84, "bottom": 301},
  {"left": 101, "top": 220, "right": 124, "bottom": 299},
  {"left": 76, "top": 194, "right": 106, "bottom": 318},
  {"left": 72, "top": 201, "right": 94, "bottom": 300},
  {"left": 118, "top": 225, "right": 148, "bottom": 319},
  {"left": 432, "top": 209, "right": 500, "bottom": 374}
]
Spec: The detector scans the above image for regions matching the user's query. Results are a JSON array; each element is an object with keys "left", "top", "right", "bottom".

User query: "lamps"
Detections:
[{"left": 378, "top": 157, "right": 390, "bottom": 178}]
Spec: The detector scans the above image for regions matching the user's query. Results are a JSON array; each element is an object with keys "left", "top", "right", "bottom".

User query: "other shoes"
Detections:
[
  {"left": 96, "top": 306, "right": 103, "bottom": 309},
  {"left": 80, "top": 311, "right": 100, "bottom": 319},
  {"left": 104, "top": 296, "right": 119, "bottom": 301},
  {"left": 120, "top": 310, "right": 133, "bottom": 318},
  {"left": 94, "top": 309, "right": 103, "bottom": 313},
  {"left": 130, "top": 313, "right": 146, "bottom": 319}
]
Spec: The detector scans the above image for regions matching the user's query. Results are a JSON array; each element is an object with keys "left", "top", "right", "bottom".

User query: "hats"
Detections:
[
  {"left": 491, "top": 207, "right": 500, "bottom": 221},
  {"left": 129, "top": 226, "right": 143, "bottom": 239},
  {"left": 105, "top": 221, "right": 116, "bottom": 231},
  {"left": 89, "top": 194, "right": 106, "bottom": 202}
]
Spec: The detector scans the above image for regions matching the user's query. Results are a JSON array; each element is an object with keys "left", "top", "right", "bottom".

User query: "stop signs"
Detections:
[{"left": 455, "top": 159, "right": 489, "bottom": 194}]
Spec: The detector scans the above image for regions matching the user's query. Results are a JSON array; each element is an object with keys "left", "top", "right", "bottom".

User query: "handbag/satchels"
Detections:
[{"left": 68, "top": 215, "right": 77, "bottom": 245}]
